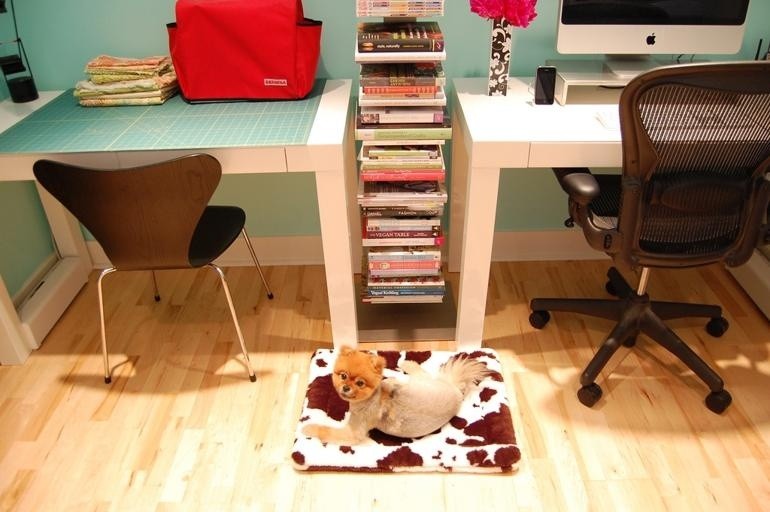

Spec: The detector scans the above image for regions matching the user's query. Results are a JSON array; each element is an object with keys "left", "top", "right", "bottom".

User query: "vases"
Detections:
[{"left": 488, "top": 18, "right": 513, "bottom": 96}]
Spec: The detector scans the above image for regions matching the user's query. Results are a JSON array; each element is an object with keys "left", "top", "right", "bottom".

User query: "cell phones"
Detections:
[{"left": 535, "top": 65, "right": 557, "bottom": 104}]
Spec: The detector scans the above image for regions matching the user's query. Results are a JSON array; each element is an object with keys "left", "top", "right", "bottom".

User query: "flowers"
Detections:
[{"left": 468, "top": 0, "right": 538, "bottom": 27}]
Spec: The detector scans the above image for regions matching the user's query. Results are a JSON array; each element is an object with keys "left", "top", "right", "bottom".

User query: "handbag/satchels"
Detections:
[{"left": 166, "top": 0, "right": 322, "bottom": 104}]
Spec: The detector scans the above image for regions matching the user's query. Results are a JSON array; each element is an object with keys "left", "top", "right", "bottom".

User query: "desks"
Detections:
[
  {"left": 447, "top": 61, "right": 770, "bottom": 352},
  {"left": 1, "top": 78, "right": 361, "bottom": 365}
]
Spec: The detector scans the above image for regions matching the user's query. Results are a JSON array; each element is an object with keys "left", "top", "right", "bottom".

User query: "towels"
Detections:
[{"left": 72, "top": 55, "right": 179, "bottom": 107}]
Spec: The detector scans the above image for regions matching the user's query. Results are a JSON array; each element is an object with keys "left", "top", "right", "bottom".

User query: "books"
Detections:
[{"left": 354, "top": 21, "right": 453, "bottom": 306}]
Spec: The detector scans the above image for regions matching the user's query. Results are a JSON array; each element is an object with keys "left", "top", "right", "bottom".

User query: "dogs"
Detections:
[{"left": 302, "top": 344, "right": 490, "bottom": 447}]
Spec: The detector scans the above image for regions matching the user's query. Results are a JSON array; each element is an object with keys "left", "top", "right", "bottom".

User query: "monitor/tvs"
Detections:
[{"left": 555, "top": 0, "right": 750, "bottom": 78}]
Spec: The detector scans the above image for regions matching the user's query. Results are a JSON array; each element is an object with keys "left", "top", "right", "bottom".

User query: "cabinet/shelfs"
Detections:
[{"left": 355, "top": 2, "right": 458, "bottom": 345}]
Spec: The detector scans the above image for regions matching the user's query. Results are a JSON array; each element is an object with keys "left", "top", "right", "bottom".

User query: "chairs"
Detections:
[
  {"left": 33, "top": 153, "right": 275, "bottom": 385},
  {"left": 528, "top": 62, "right": 770, "bottom": 414}
]
